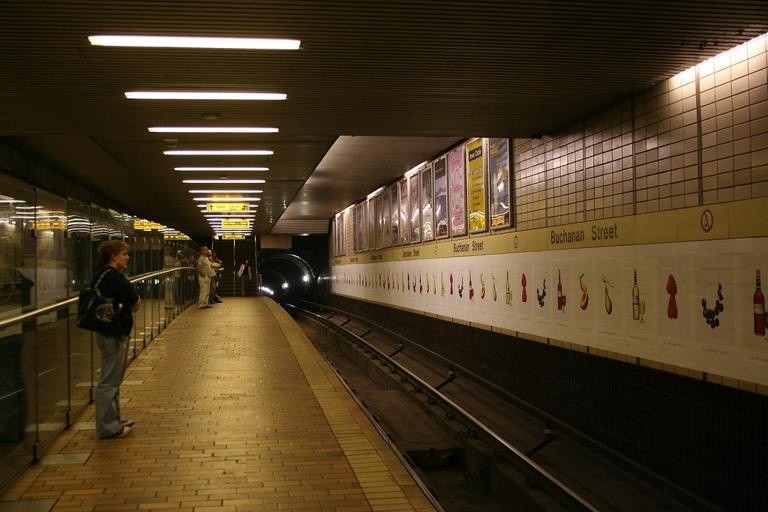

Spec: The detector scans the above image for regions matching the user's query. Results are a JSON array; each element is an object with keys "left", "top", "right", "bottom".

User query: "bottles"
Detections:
[
  {"left": 752, "top": 268, "right": 766, "bottom": 337},
  {"left": 631, "top": 270, "right": 640, "bottom": 321},
  {"left": 557, "top": 268, "right": 563, "bottom": 310},
  {"left": 505, "top": 269, "right": 511, "bottom": 305}
]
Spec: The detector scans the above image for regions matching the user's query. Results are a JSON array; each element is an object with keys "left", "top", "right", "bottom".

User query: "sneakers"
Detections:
[
  {"left": 200, "top": 298, "right": 222, "bottom": 308},
  {"left": 100, "top": 421, "right": 135, "bottom": 438}
]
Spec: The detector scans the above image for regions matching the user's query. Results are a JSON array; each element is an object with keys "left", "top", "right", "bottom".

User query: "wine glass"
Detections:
[
  {"left": 561, "top": 295, "right": 567, "bottom": 314},
  {"left": 638, "top": 301, "right": 646, "bottom": 324}
]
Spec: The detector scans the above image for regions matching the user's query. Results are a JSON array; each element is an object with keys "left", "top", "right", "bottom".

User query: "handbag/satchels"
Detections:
[{"left": 76, "top": 288, "right": 125, "bottom": 335}]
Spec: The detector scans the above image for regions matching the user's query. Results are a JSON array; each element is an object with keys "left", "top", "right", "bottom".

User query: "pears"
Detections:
[
  {"left": 605, "top": 286, "right": 612, "bottom": 314},
  {"left": 492, "top": 283, "right": 497, "bottom": 301}
]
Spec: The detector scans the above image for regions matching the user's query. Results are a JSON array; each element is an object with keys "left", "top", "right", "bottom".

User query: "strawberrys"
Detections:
[
  {"left": 522, "top": 287, "right": 527, "bottom": 302},
  {"left": 666, "top": 274, "right": 678, "bottom": 293},
  {"left": 668, "top": 296, "right": 677, "bottom": 319},
  {"left": 522, "top": 273, "right": 527, "bottom": 287}
]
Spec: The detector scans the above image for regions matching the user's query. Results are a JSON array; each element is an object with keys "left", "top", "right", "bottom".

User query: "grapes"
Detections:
[
  {"left": 537, "top": 279, "right": 546, "bottom": 308},
  {"left": 702, "top": 284, "right": 725, "bottom": 329}
]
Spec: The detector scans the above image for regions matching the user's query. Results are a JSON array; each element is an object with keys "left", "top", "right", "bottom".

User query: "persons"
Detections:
[
  {"left": 237, "top": 255, "right": 253, "bottom": 297},
  {"left": 196, "top": 245, "right": 223, "bottom": 310},
  {"left": 86, "top": 237, "right": 142, "bottom": 443},
  {"left": 161, "top": 245, "right": 197, "bottom": 311}
]
